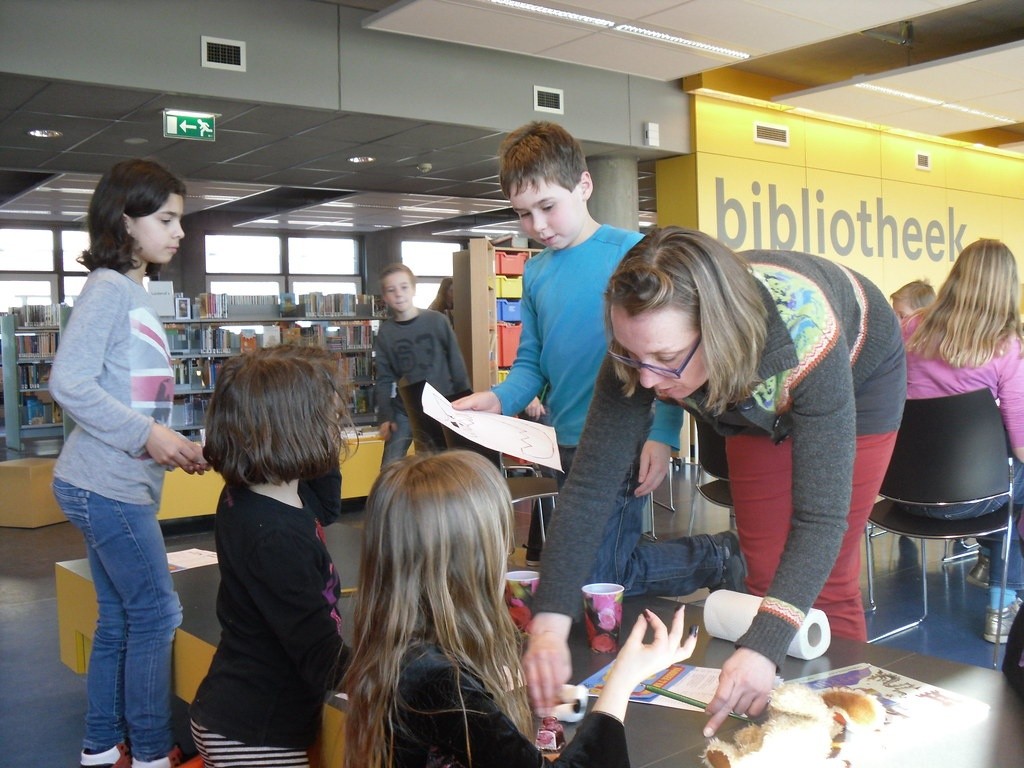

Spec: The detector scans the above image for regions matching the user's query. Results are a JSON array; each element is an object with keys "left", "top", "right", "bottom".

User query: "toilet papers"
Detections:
[
  {"left": 703, "top": 588, "right": 831, "bottom": 662},
  {"left": 552, "top": 684, "right": 589, "bottom": 722}
]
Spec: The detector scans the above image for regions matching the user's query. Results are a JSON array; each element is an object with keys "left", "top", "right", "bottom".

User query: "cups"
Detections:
[
  {"left": 582, "top": 583, "right": 624, "bottom": 652},
  {"left": 505, "top": 571, "right": 540, "bottom": 630}
]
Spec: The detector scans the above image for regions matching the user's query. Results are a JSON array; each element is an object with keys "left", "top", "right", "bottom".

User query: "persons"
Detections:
[
  {"left": 428, "top": 278, "right": 453, "bottom": 314},
  {"left": 376, "top": 263, "right": 472, "bottom": 473},
  {"left": 451, "top": 121, "right": 907, "bottom": 737},
  {"left": 346, "top": 450, "right": 700, "bottom": 768},
  {"left": 891, "top": 239, "right": 1024, "bottom": 643},
  {"left": 48, "top": 159, "right": 212, "bottom": 768},
  {"left": 188, "top": 344, "right": 363, "bottom": 768}
]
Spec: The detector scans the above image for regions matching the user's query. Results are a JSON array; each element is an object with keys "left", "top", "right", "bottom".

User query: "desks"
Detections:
[{"left": 56, "top": 523, "right": 1024, "bottom": 768}]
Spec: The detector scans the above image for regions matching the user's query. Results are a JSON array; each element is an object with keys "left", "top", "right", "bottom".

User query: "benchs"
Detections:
[{"left": 156, "top": 441, "right": 415, "bottom": 520}]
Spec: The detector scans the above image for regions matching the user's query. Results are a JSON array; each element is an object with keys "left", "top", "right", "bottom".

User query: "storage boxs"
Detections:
[{"left": 0, "top": 456, "right": 69, "bottom": 528}]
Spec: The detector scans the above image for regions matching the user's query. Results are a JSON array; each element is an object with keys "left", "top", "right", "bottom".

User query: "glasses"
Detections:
[{"left": 607, "top": 330, "right": 702, "bottom": 379}]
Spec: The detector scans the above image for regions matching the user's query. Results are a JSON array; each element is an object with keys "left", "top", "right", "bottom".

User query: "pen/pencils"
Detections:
[{"left": 639, "top": 682, "right": 757, "bottom": 723}]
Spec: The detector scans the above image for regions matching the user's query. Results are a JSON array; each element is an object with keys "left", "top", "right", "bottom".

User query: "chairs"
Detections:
[
  {"left": 506, "top": 476, "right": 559, "bottom": 545},
  {"left": 687, "top": 417, "right": 734, "bottom": 538},
  {"left": 863, "top": 387, "right": 1016, "bottom": 667}
]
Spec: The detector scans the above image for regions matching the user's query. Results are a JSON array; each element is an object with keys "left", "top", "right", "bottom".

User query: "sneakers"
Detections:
[
  {"left": 967, "top": 553, "right": 991, "bottom": 589},
  {"left": 707, "top": 529, "right": 748, "bottom": 595},
  {"left": 985, "top": 597, "right": 1023, "bottom": 644},
  {"left": 132, "top": 745, "right": 185, "bottom": 768},
  {"left": 81, "top": 738, "right": 132, "bottom": 768}
]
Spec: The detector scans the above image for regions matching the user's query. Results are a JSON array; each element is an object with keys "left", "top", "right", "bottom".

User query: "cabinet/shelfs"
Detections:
[
  {"left": 0, "top": 291, "right": 387, "bottom": 459},
  {"left": 453, "top": 239, "right": 545, "bottom": 392}
]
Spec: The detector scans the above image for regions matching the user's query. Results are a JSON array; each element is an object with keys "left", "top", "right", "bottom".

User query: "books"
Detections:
[{"left": 1, "top": 280, "right": 387, "bottom": 427}]
